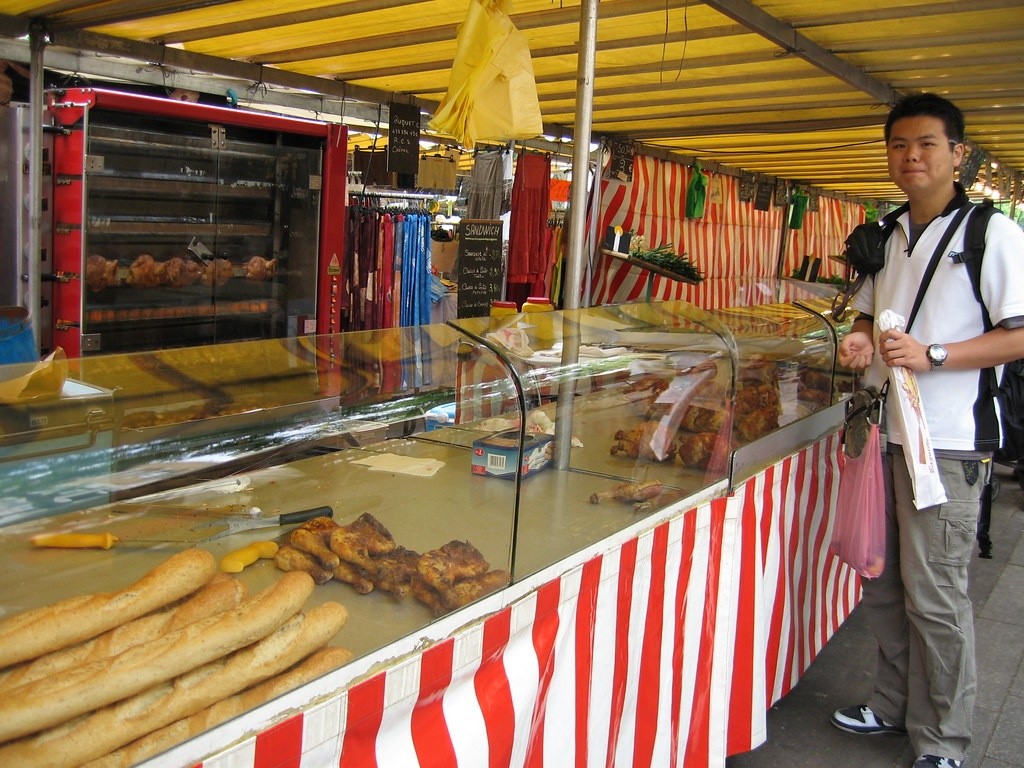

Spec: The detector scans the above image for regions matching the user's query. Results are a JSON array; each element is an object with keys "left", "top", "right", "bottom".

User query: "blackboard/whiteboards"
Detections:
[
  {"left": 457, "top": 218, "right": 503, "bottom": 320},
  {"left": 387, "top": 100, "right": 422, "bottom": 175}
]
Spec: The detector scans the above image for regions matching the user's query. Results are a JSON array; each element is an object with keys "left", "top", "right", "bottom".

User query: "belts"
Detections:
[{"left": 963, "top": 459, "right": 979, "bottom": 485}]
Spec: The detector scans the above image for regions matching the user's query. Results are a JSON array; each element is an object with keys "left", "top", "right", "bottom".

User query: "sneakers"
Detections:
[
  {"left": 912, "top": 754, "right": 965, "bottom": 768},
  {"left": 830, "top": 704, "right": 909, "bottom": 736}
]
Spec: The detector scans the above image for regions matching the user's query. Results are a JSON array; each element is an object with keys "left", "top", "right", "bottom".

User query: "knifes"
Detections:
[{"left": 28, "top": 532, "right": 199, "bottom": 544}]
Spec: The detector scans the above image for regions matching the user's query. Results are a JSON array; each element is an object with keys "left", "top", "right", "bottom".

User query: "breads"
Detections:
[{"left": 0, "top": 548, "right": 353, "bottom": 768}]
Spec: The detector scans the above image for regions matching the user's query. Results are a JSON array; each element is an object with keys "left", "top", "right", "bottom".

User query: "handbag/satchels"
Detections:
[
  {"left": 844, "top": 385, "right": 887, "bottom": 459},
  {"left": 829, "top": 424, "right": 886, "bottom": 579}
]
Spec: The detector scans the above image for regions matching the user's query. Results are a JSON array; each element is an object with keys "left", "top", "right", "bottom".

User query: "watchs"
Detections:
[{"left": 926, "top": 343, "right": 947, "bottom": 371}]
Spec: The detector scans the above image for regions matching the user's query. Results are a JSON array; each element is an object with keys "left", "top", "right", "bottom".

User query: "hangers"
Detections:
[{"left": 347, "top": 192, "right": 432, "bottom": 219}]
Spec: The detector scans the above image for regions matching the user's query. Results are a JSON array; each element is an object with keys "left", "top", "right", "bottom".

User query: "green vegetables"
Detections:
[{"left": 634, "top": 240, "right": 705, "bottom": 282}]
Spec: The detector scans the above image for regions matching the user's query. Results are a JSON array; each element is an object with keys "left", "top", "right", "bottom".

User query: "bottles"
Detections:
[
  {"left": 522, "top": 297, "right": 555, "bottom": 349},
  {"left": 489, "top": 301, "right": 518, "bottom": 333},
  {"left": 590, "top": 478, "right": 662, "bottom": 504}
]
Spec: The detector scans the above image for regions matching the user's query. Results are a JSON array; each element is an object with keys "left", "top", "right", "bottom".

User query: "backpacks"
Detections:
[{"left": 873, "top": 197, "right": 1024, "bottom": 463}]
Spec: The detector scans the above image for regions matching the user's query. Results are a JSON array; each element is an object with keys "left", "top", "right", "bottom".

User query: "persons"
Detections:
[{"left": 828, "top": 93, "right": 1024, "bottom": 768}]
